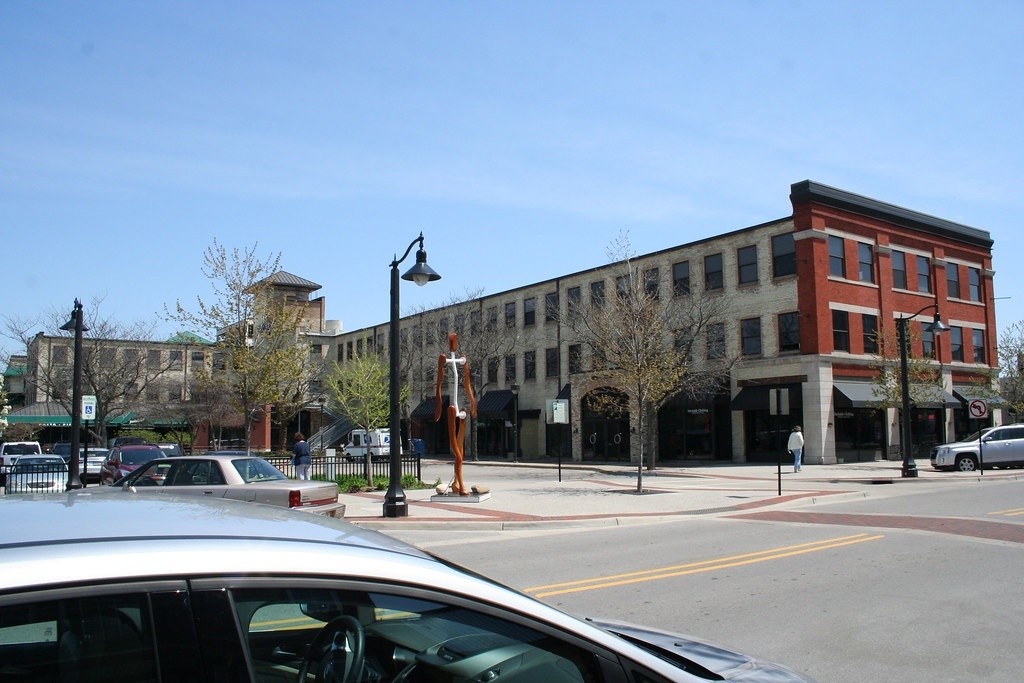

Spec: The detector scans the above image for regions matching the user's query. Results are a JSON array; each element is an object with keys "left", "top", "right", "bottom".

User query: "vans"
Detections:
[{"left": 343, "top": 428, "right": 403, "bottom": 463}]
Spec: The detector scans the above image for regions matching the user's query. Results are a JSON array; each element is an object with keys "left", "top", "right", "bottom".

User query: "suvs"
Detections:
[{"left": 931, "top": 423, "right": 1024, "bottom": 473}]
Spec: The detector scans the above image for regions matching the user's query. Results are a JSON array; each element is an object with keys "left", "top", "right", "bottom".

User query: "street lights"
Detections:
[
  {"left": 58, "top": 297, "right": 89, "bottom": 489},
  {"left": 892, "top": 303, "right": 950, "bottom": 477},
  {"left": 384, "top": 230, "right": 441, "bottom": 516}
]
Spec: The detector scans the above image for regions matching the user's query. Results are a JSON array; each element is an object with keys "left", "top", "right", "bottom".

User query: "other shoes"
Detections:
[{"left": 793, "top": 468, "right": 802, "bottom": 473}]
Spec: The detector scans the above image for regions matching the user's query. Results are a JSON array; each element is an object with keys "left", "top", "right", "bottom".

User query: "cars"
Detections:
[
  {"left": 70, "top": 455, "right": 346, "bottom": 520},
  {"left": 0, "top": 438, "right": 183, "bottom": 497},
  {"left": 2, "top": 499, "right": 807, "bottom": 683}
]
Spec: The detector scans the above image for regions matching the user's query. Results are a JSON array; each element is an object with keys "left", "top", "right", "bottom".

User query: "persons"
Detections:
[
  {"left": 787, "top": 425, "right": 805, "bottom": 473},
  {"left": 291, "top": 433, "right": 313, "bottom": 480},
  {"left": 434, "top": 333, "right": 477, "bottom": 496}
]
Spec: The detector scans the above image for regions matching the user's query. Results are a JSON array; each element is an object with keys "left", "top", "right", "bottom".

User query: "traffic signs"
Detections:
[{"left": 968, "top": 398, "right": 989, "bottom": 419}]
